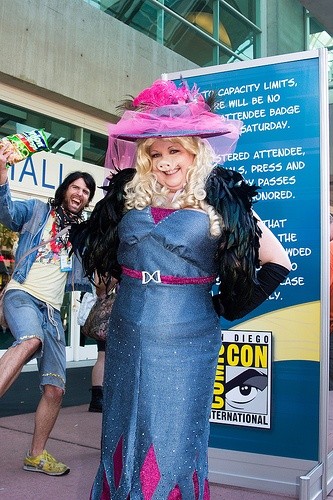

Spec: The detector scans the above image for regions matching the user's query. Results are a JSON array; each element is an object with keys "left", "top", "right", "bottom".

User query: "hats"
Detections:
[{"left": 104, "top": 80, "right": 245, "bottom": 172}]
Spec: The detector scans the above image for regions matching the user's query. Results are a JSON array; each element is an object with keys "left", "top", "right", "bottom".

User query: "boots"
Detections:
[{"left": 88, "top": 386, "right": 103, "bottom": 413}]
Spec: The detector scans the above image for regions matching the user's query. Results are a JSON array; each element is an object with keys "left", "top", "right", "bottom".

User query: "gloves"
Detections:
[{"left": 212, "top": 262, "right": 290, "bottom": 322}]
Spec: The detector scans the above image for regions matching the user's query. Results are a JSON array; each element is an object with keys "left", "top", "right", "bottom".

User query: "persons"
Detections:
[
  {"left": 66, "top": 77, "right": 293, "bottom": 500},
  {"left": 87, "top": 269, "right": 119, "bottom": 414},
  {"left": 0, "top": 142, "right": 95, "bottom": 477}
]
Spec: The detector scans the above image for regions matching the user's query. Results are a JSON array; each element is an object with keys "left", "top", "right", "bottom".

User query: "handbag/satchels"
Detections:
[
  {"left": 81, "top": 294, "right": 114, "bottom": 341},
  {"left": 0, "top": 284, "right": 7, "bottom": 327}
]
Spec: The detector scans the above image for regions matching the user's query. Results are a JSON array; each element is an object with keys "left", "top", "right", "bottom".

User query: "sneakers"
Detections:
[{"left": 23, "top": 449, "right": 70, "bottom": 476}]
